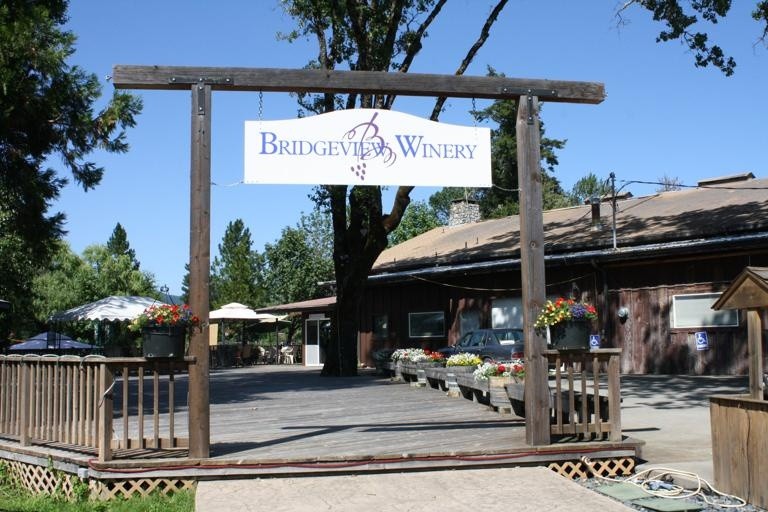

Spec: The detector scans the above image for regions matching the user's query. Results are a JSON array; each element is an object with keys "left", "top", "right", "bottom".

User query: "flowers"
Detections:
[
  {"left": 533, "top": 295, "right": 598, "bottom": 329},
  {"left": 127, "top": 304, "right": 207, "bottom": 349},
  {"left": 391, "top": 348, "right": 525, "bottom": 381}
]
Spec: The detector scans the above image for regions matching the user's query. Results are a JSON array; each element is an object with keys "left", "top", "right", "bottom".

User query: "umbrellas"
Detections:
[
  {"left": 2, "top": 327, "right": 106, "bottom": 352},
  {"left": 235, "top": 312, "right": 292, "bottom": 339},
  {"left": 207, "top": 301, "right": 278, "bottom": 344}
]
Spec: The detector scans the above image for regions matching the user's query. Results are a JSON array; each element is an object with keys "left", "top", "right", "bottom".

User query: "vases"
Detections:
[
  {"left": 489, "top": 376, "right": 522, "bottom": 407},
  {"left": 447, "top": 366, "right": 477, "bottom": 398},
  {"left": 142, "top": 326, "right": 186, "bottom": 358},
  {"left": 549, "top": 320, "right": 591, "bottom": 351}
]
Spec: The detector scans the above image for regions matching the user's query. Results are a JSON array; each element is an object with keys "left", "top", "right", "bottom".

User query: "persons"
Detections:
[{"left": 230, "top": 339, "right": 298, "bottom": 365}]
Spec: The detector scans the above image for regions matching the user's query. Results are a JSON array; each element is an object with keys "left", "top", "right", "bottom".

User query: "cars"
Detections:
[{"left": 438, "top": 329, "right": 524, "bottom": 364}]
